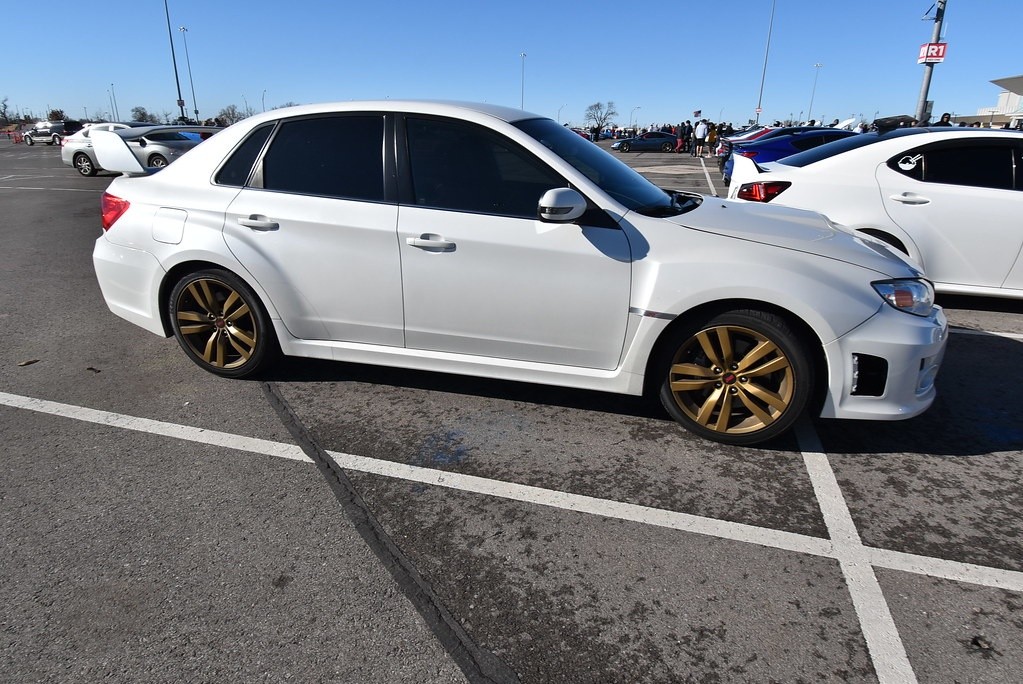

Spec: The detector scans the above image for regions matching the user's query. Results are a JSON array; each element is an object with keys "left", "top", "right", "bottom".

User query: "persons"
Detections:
[
  {"left": 590, "top": 123, "right": 601, "bottom": 142},
  {"left": 647, "top": 120, "right": 693, "bottom": 153},
  {"left": 807, "top": 120, "right": 816, "bottom": 127},
  {"left": 610, "top": 126, "right": 647, "bottom": 139},
  {"left": 931, "top": 112, "right": 952, "bottom": 127},
  {"left": 689, "top": 119, "right": 733, "bottom": 159},
  {"left": 969, "top": 122, "right": 981, "bottom": 128},
  {"left": 852, "top": 123, "right": 877, "bottom": 134},
  {"left": 829, "top": 119, "right": 839, "bottom": 127},
  {"left": 910, "top": 113, "right": 931, "bottom": 127},
  {"left": 958, "top": 122, "right": 967, "bottom": 127}
]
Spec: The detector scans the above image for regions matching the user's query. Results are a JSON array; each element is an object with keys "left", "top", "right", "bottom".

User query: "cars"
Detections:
[
  {"left": 572, "top": 127, "right": 610, "bottom": 141},
  {"left": 728, "top": 128, "right": 1023, "bottom": 301},
  {"left": 722, "top": 130, "right": 862, "bottom": 187},
  {"left": 713, "top": 124, "right": 783, "bottom": 175},
  {"left": 610, "top": 131, "right": 678, "bottom": 153},
  {"left": 94, "top": 101, "right": 947, "bottom": 448},
  {"left": 61, "top": 123, "right": 200, "bottom": 177}
]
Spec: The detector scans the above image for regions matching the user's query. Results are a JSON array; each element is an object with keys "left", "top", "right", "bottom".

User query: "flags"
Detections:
[{"left": 693, "top": 110, "right": 702, "bottom": 117}]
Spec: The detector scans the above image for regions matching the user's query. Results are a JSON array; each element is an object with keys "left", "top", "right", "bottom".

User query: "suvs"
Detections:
[{"left": 22, "top": 120, "right": 84, "bottom": 146}]
